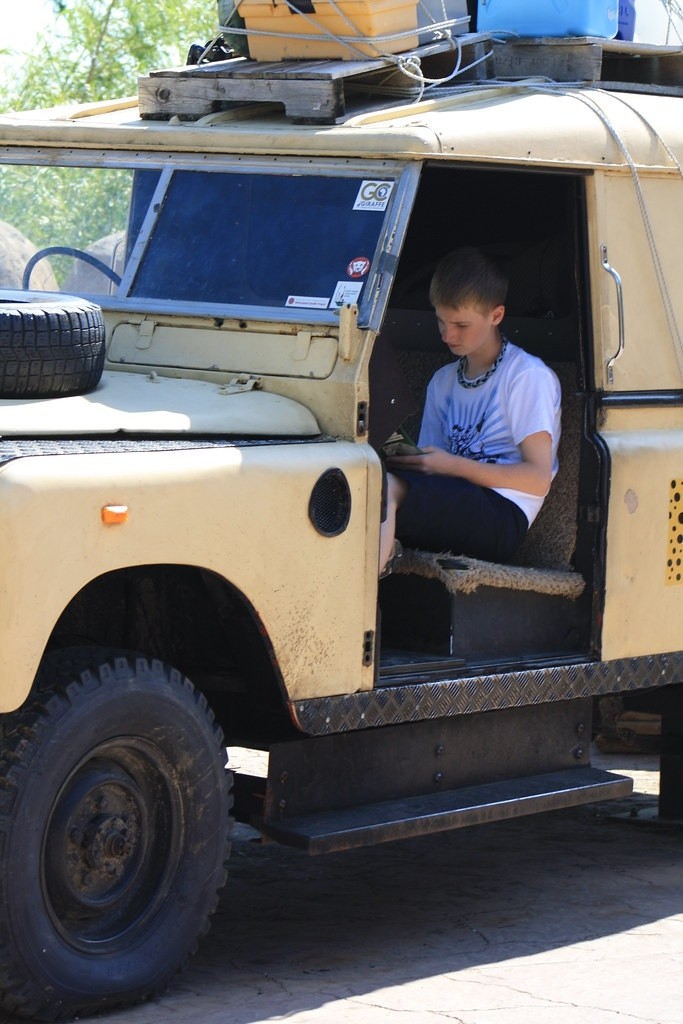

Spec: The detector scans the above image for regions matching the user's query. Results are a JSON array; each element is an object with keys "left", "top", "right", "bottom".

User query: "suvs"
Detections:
[{"left": 2, "top": 0, "right": 683, "bottom": 1024}]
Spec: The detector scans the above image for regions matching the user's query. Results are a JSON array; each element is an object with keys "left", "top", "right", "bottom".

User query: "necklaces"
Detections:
[{"left": 457, "top": 334, "right": 508, "bottom": 389}]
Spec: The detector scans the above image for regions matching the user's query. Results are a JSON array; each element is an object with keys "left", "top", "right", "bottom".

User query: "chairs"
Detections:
[{"left": 386, "top": 357, "right": 586, "bottom": 662}]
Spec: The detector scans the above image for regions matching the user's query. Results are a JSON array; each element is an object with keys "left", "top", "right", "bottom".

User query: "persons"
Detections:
[{"left": 379, "top": 237, "right": 562, "bottom": 568}]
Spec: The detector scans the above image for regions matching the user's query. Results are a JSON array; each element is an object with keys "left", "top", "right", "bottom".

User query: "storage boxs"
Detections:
[
  {"left": 233, "top": 1, "right": 421, "bottom": 65},
  {"left": 476, "top": 0, "right": 620, "bottom": 39}
]
